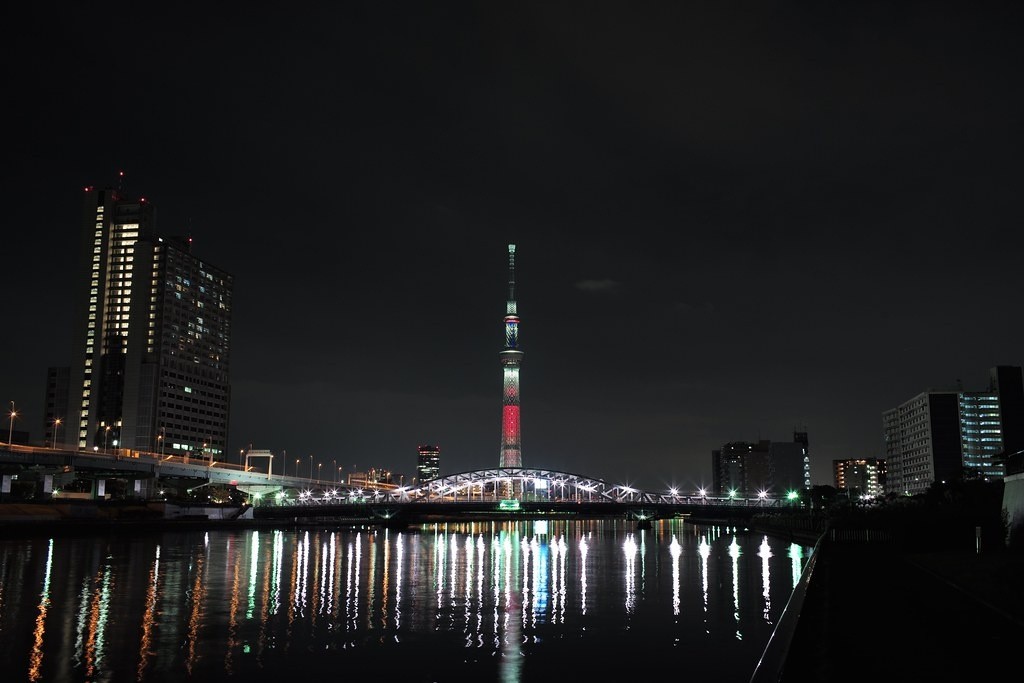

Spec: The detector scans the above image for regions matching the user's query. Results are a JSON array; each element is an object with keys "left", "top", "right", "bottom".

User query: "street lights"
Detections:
[
  {"left": 54, "top": 419, "right": 60, "bottom": 450},
  {"left": 104, "top": 426, "right": 111, "bottom": 454},
  {"left": 157, "top": 436, "right": 162, "bottom": 458},
  {"left": 758, "top": 490, "right": 768, "bottom": 507},
  {"left": 624, "top": 485, "right": 630, "bottom": 502},
  {"left": 699, "top": 489, "right": 708, "bottom": 505},
  {"left": 670, "top": 487, "right": 679, "bottom": 504},
  {"left": 9, "top": 412, "right": 16, "bottom": 444},
  {"left": 11, "top": 401, "right": 15, "bottom": 412},
  {"left": 787, "top": 490, "right": 798, "bottom": 507},
  {"left": 162, "top": 426, "right": 166, "bottom": 460},
  {"left": 729, "top": 489, "right": 738, "bottom": 505},
  {"left": 201, "top": 436, "right": 416, "bottom": 488}
]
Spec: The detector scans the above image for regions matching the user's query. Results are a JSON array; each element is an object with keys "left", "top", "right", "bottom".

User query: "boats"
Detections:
[
  {"left": 637, "top": 510, "right": 652, "bottom": 530},
  {"left": 684, "top": 506, "right": 753, "bottom": 535},
  {"left": 365, "top": 519, "right": 409, "bottom": 534},
  {"left": 625, "top": 512, "right": 637, "bottom": 521},
  {"left": 650, "top": 511, "right": 660, "bottom": 520}
]
